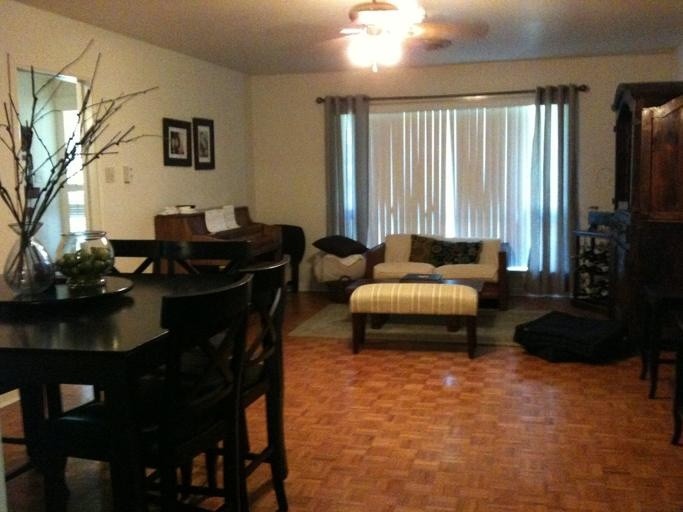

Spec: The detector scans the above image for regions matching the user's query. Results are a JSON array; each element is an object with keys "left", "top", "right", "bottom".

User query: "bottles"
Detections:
[{"left": 569, "top": 245, "right": 608, "bottom": 302}]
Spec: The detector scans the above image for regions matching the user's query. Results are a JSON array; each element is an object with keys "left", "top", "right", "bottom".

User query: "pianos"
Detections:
[{"left": 154, "top": 204, "right": 302, "bottom": 291}]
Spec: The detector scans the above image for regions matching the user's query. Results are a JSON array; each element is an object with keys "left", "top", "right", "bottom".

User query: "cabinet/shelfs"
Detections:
[{"left": 610, "top": 80, "right": 683, "bottom": 220}]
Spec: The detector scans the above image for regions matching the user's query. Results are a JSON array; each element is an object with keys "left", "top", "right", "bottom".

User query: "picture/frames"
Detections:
[
  {"left": 162, "top": 117, "right": 192, "bottom": 167},
  {"left": 192, "top": 117, "right": 215, "bottom": 170}
]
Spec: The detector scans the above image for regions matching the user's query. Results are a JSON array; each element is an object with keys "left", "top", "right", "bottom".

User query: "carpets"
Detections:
[{"left": 289, "top": 302, "right": 549, "bottom": 347}]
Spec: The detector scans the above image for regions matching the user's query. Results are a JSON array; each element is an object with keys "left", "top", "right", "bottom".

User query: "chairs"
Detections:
[
  {"left": 155, "top": 240, "right": 249, "bottom": 273},
  {"left": 236, "top": 254, "right": 288, "bottom": 512},
  {"left": 109, "top": 240, "right": 155, "bottom": 274},
  {"left": 49, "top": 273, "right": 254, "bottom": 512}
]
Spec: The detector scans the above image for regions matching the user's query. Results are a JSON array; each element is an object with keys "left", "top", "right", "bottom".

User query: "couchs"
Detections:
[{"left": 367, "top": 234, "right": 508, "bottom": 310}]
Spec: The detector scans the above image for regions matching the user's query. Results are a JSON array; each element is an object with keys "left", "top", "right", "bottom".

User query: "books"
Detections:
[{"left": 400, "top": 273, "right": 442, "bottom": 283}]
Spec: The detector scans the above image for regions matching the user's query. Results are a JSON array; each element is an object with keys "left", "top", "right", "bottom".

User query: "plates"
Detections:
[{"left": 163, "top": 206, "right": 197, "bottom": 214}]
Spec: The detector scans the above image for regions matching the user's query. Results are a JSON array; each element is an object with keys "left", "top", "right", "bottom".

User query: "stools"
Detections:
[
  {"left": 314, "top": 253, "right": 367, "bottom": 303},
  {"left": 349, "top": 283, "right": 479, "bottom": 359}
]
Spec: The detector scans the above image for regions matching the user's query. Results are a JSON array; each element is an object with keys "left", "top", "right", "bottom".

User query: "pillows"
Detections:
[
  {"left": 445, "top": 241, "right": 482, "bottom": 264},
  {"left": 409, "top": 234, "right": 444, "bottom": 268},
  {"left": 313, "top": 235, "right": 367, "bottom": 258}
]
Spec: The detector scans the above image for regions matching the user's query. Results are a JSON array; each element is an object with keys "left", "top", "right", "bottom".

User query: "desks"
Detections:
[
  {"left": 0, "top": 274, "right": 242, "bottom": 512},
  {"left": 640, "top": 285, "right": 683, "bottom": 398},
  {"left": 344, "top": 278, "right": 486, "bottom": 329}
]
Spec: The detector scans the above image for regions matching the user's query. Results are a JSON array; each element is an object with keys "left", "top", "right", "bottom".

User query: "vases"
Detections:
[
  {"left": 55, "top": 231, "right": 115, "bottom": 291},
  {"left": 3, "top": 222, "right": 55, "bottom": 296}
]
